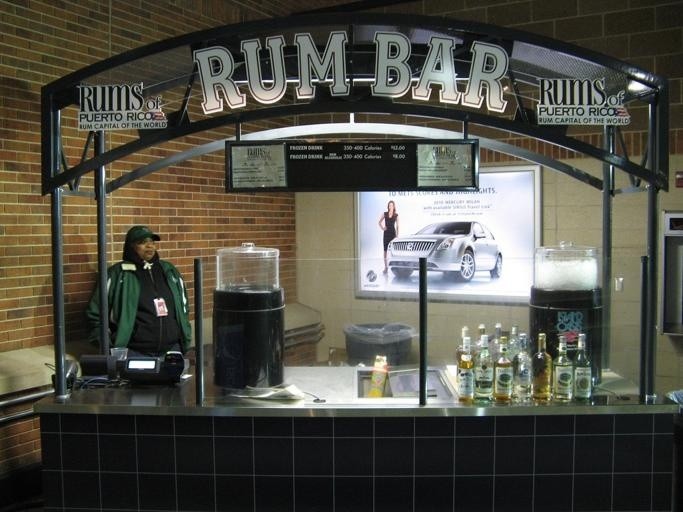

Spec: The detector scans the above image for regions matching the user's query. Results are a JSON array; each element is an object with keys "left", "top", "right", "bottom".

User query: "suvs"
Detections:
[{"left": 385, "top": 220, "right": 504, "bottom": 284}]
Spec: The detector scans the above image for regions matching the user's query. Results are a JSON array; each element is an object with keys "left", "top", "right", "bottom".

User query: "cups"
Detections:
[{"left": 109, "top": 347, "right": 129, "bottom": 360}]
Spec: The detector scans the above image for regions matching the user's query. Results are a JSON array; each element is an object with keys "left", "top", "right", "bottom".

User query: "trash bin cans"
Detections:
[{"left": 342, "top": 323, "right": 413, "bottom": 365}]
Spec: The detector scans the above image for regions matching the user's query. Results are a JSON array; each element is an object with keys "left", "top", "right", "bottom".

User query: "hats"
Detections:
[{"left": 126, "top": 226, "right": 160, "bottom": 243}]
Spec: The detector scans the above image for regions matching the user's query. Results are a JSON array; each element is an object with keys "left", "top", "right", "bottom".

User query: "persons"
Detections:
[
  {"left": 85, "top": 226, "right": 193, "bottom": 362},
  {"left": 378, "top": 200, "right": 400, "bottom": 274}
]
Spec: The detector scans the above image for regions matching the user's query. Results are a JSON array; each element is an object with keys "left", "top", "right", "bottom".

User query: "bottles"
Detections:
[{"left": 455, "top": 323, "right": 592, "bottom": 405}]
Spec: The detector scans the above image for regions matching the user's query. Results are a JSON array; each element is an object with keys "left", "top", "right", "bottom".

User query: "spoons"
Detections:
[{"left": 303, "top": 391, "right": 326, "bottom": 402}]
